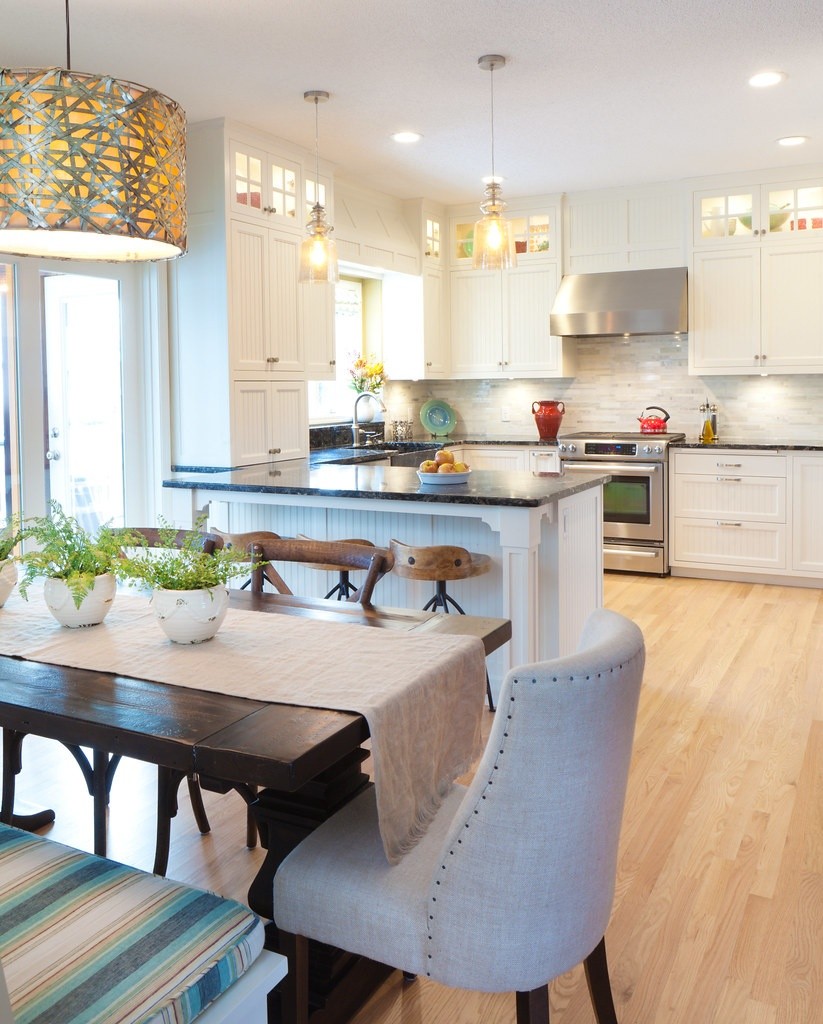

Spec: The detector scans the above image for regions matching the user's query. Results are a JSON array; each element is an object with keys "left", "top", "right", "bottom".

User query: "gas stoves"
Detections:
[{"left": 557, "top": 430, "right": 687, "bottom": 463}]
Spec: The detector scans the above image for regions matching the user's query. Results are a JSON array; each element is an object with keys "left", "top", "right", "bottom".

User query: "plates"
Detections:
[{"left": 419, "top": 399, "right": 457, "bottom": 435}]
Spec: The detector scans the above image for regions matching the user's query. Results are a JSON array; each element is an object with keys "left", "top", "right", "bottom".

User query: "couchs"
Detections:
[{"left": 1, "top": 819, "right": 289, "bottom": 1022}]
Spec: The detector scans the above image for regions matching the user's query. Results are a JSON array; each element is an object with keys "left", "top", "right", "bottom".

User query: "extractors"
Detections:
[{"left": 550, "top": 267, "right": 690, "bottom": 341}]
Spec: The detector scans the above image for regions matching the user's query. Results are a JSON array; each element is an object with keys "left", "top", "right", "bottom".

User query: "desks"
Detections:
[
  {"left": 1, "top": 577, "right": 514, "bottom": 876},
  {"left": 161, "top": 442, "right": 612, "bottom": 704}
]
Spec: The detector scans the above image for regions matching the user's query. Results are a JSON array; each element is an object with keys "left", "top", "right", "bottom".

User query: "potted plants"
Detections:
[{"left": 0, "top": 495, "right": 271, "bottom": 645}]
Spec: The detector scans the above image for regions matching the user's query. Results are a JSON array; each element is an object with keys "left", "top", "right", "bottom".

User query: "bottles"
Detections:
[{"left": 699, "top": 404, "right": 719, "bottom": 438}]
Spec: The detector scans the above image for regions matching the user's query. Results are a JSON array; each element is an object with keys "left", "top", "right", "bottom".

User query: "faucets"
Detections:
[
  {"left": 350, "top": 391, "right": 388, "bottom": 448},
  {"left": 365, "top": 432, "right": 383, "bottom": 444}
]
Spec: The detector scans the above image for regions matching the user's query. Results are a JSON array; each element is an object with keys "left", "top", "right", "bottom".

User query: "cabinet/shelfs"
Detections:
[
  {"left": 229, "top": 220, "right": 336, "bottom": 467},
  {"left": 685, "top": 179, "right": 822, "bottom": 375},
  {"left": 669, "top": 449, "right": 823, "bottom": 586},
  {"left": 383, "top": 198, "right": 578, "bottom": 381}
]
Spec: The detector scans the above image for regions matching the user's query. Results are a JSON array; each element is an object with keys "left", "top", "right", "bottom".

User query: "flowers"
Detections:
[{"left": 345, "top": 351, "right": 385, "bottom": 399}]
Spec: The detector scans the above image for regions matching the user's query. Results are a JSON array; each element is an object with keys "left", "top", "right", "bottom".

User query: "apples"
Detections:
[{"left": 419, "top": 450, "right": 470, "bottom": 473}]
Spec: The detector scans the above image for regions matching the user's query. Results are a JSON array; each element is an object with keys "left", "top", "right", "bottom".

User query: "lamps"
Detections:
[
  {"left": 475, "top": 54, "right": 517, "bottom": 274},
  {"left": 300, "top": 91, "right": 332, "bottom": 273},
  {"left": 0, "top": 0, "right": 187, "bottom": 265}
]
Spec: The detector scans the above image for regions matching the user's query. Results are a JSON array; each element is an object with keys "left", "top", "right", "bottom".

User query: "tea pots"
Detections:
[{"left": 637, "top": 406, "right": 670, "bottom": 434}]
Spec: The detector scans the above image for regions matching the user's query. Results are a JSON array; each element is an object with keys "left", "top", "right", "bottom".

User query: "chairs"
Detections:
[
  {"left": 63, "top": 528, "right": 472, "bottom": 876},
  {"left": 248, "top": 616, "right": 645, "bottom": 1023}
]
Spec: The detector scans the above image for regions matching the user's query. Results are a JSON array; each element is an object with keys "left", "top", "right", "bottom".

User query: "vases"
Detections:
[{"left": 350, "top": 393, "right": 373, "bottom": 425}]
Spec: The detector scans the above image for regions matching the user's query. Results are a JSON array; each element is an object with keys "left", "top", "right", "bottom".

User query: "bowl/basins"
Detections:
[
  {"left": 515, "top": 241, "right": 528, "bottom": 252},
  {"left": 790, "top": 217, "right": 823, "bottom": 231},
  {"left": 739, "top": 212, "right": 792, "bottom": 233}
]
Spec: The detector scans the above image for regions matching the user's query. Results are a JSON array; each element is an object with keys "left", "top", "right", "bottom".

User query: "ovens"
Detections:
[{"left": 558, "top": 457, "right": 671, "bottom": 578}]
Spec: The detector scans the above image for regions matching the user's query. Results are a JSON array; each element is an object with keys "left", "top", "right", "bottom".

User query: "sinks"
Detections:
[{"left": 347, "top": 445, "right": 442, "bottom": 467}]
